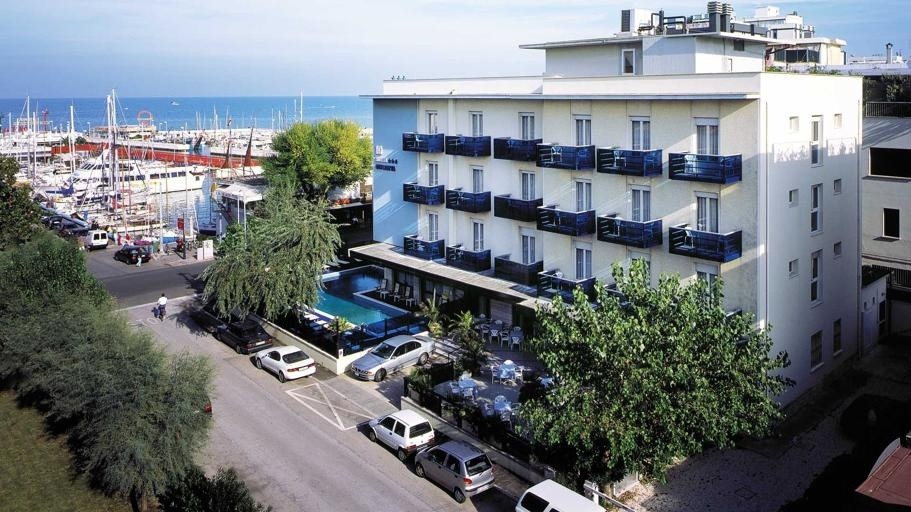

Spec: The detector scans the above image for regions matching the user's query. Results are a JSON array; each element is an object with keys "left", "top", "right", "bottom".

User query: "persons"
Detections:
[
  {"left": 157, "top": 293, "right": 167, "bottom": 322},
  {"left": 118, "top": 232, "right": 130, "bottom": 246},
  {"left": 164, "top": 243, "right": 170, "bottom": 253},
  {"left": 135, "top": 250, "right": 142, "bottom": 267}
]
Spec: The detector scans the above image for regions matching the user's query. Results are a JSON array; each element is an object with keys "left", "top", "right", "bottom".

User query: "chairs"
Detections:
[
  {"left": 550, "top": 147, "right": 563, "bottom": 163},
  {"left": 448, "top": 359, "right": 526, "bottom": 425},
  {"left": 613, "top": 217, "right": 624, "bottom": 237},
  {"left": 375, "top": 278, "right": 412, "bottom": 304},
  {"left": 684, "top": 225, "right": 698, "bottom": 247},
  {"left": 614, "top": 149, "right": 627, "bottom": 167},
  {"left": 683, "top": 155, "right": 698, "bottom": 173},
  {"left": 473, "top": 314, "right": 527, "bottom": 352},
  {"left": 549, "top": 271, "right": 564, "bottom": 290}
]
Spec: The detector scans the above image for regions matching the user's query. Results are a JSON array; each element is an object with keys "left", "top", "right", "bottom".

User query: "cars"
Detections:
[
  {"left": 115, "top": 241, "right": 151, "bottom": 264},
  {"left": 76, "top": 230, "right": 109, "bottom": 251},
  {"left": 351, "top": 334, "right": 436, "bottom": 382},
  {"left": 211, "top": 318, "right": 316, "bottom": 382},
  {"left": 369, "top": 409, "right": 496, "bottom": 503}
]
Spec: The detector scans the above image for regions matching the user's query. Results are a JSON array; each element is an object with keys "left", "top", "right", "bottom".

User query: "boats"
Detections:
[
  {"left": 0, "top": 89, "right": 208, "bottom": 227},
  {"left": 106, "top": 223, "right": 197, "bottom": 252},
  {"left": 206, "top": 98, "right": 272, "bottom": 159}
]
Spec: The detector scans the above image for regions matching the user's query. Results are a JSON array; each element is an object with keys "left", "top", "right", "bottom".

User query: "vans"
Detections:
[{"left": 515, "top": 479, "right": 608, "bottom": 512}]
